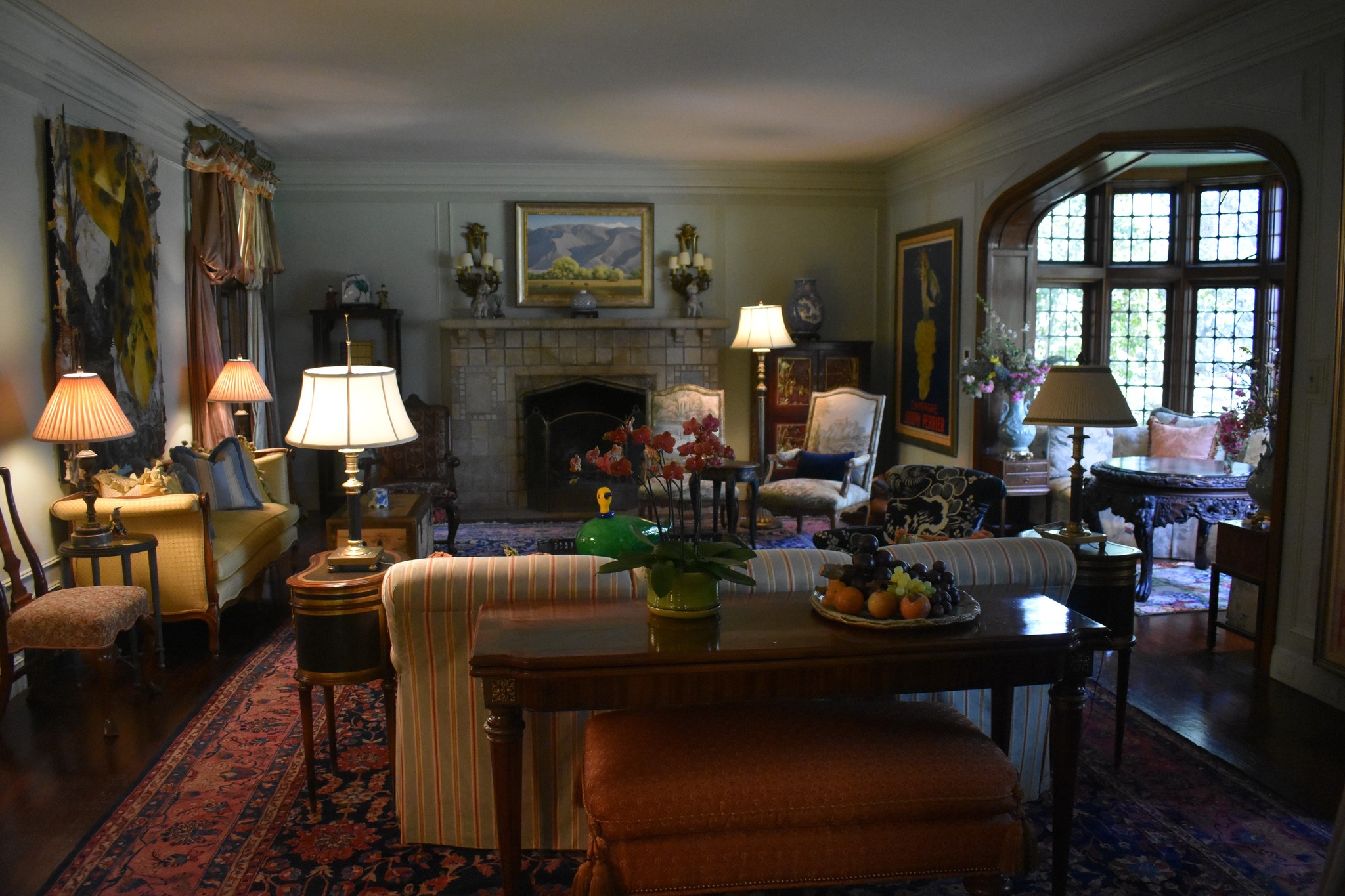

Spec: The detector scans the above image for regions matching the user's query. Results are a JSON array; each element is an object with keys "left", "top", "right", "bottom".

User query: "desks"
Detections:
[{"left": 59, "top": 458, "right": 1268, "bottom": 896}]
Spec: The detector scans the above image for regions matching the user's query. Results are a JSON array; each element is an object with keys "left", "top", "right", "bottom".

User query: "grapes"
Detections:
[{"left": 844, "top": 533, "right": 961, "bottom": 618}]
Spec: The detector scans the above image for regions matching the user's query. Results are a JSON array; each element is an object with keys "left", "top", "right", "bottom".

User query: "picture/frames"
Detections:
[
  {"left": 515, "top": 201, "right": 654, "bottom": 308},
  {"left": 894, "top": 216, "right": 962, "bottom": 458}
]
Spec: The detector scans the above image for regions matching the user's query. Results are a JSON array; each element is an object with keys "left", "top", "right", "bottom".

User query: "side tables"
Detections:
[{"left": 983, "top": 458, "right": 1052, "bottom": 539}]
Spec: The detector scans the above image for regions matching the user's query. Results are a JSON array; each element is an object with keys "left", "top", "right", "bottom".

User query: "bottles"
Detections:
[
  {"left": 570, "top": 289, "right": 597, "bottom": 310},
  {"left": 785, "top": 277, "right": 825, "bottom": 334}
]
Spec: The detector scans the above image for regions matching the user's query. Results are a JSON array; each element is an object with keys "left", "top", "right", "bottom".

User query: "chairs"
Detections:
[
  {"left": 0, "top": 467, "right": 150, "bottom": 747},
  {"left": 360, "top": 384, "right": 1007, "bottom": 552}
]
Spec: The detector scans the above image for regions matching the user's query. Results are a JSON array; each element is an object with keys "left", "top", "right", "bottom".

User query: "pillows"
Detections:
[
  {"left": 1146, "top": 416, "right": 1222, "bottom": 460},
  {"left": 92, "top": 433, "right": 277, "bottom": 541},
  {"left": 794, "top": 450, "right": 855, "bottom": 483},
  {"left": 1046, "top": 425, "right": 1115, "bottom": 479}
]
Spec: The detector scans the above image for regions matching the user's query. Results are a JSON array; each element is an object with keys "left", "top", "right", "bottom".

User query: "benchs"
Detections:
[{"left": 49, "top": 448, "right": 301, "bottom": 662}]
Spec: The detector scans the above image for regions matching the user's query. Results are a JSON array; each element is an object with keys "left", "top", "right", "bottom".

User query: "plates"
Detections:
[{"left": 810, "top": 590, "right": 981, "bottom": 631}]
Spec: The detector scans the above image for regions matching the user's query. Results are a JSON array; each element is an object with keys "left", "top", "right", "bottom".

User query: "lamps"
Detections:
[
  {"left": 1021, "top": 364, "right": 1138, "bottom": 552},
  {"left": 453, "top": 223, "right": 713, "bottom": 318},
  {"left": 207, "top": 314, "right": 420, "bottom": 573},
  {"left": 729, "top": 299, "right": 798, "bottom": 529},
  {"left": 33, "top": 366, "right": 135, "bottom": 547}
]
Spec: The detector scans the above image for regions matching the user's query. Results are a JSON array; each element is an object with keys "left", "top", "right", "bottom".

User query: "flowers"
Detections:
[
  {"left": 960, "top": 294, "right": 1281, "bottom": 475},
  {"left": 568, "top": 414, "right": 758, "bottom": 601}
]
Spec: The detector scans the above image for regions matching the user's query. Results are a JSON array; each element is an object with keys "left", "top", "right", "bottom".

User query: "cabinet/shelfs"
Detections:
[{"left": 764, "top": 340, "right": 875, "bottom": 457}]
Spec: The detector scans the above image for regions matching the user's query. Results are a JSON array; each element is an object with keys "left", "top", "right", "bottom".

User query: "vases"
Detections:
[
  {"left": 648, "top": 571, "right": 720, "bottom": 611},
  {"left": 1245, "top": 424, "right": 1278, "bottom": 529},
  {"left": 568, "top": 275, "right": 825, "bottom": 342}
]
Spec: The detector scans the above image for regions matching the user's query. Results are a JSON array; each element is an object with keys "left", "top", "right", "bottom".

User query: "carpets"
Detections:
[{"left": 34, "top": 515, "right": 1335, "bottom": 896}]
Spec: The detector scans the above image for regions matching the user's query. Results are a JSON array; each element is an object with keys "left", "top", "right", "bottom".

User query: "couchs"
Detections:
[{"left": 381, "top": 536, "right": 1077, "bottom": 850}]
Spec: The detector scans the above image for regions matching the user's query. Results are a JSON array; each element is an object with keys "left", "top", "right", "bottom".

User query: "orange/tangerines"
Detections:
[
  {"left": 900, "top": 592, "right": 931, "bottom": 620},
  {"left": 867, "top": 589, "right": 898, "bottom": 618},
  {"left": 822, "top": 580, "right": 865, "bottom": 614}
]
